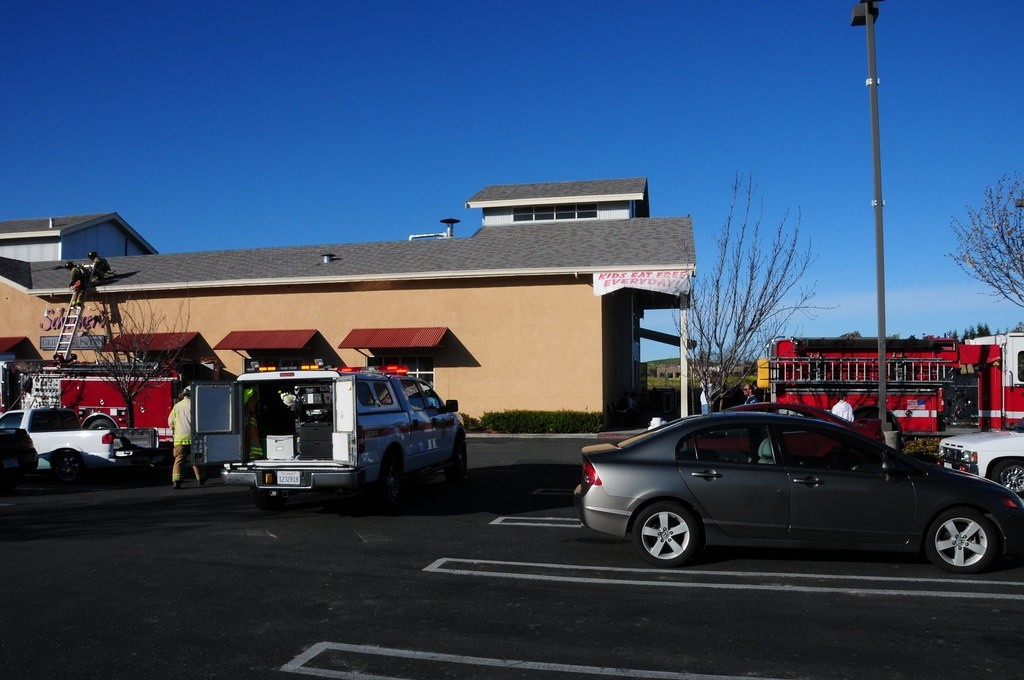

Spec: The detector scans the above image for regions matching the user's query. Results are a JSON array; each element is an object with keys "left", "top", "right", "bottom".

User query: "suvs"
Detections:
[{"left": 937, "top": 415, "right": 1024, "bottom": 500}]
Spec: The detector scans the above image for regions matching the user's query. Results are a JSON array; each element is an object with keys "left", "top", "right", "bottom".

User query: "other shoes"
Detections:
[
  {"left": 198, "top": 477, "right": 208, "bottom": 485},
  {"left": 174, "top": 482, "right": 181, "bottom": 489}
]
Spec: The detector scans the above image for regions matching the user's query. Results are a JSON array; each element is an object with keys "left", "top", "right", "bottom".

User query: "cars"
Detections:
[
  {"left": 0, "top": 427, "right": 38, "bottom": 492},
  {"left": 683, "top": 402, "right": 883, "bottom": 465},
  {"left": 573, "top": 410, "right": 1024, "bottom": 574}
]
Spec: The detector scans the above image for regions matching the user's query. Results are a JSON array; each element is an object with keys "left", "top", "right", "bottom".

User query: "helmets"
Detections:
[
  {"left": 178, "top": 386, "right": 190, "bottom": 398},
  {"left": 88, "top": 251, "right": 96, "bottom": 259},
  {"left": 65, "top": 262, "right": 73, "bottom": 268}
]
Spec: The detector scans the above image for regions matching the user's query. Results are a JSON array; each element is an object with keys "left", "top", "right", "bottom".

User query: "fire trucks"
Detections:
[
  {"left": 757, "top": 332, "right": 1024, "bottom": 436},
  {"left": 0, "top": 359, "right": 197, "bottom": 460}
]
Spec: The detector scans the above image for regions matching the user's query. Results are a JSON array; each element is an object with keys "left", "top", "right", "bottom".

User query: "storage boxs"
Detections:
[{"left": 266, "top": 435, "right": 295, "bottom": 460}]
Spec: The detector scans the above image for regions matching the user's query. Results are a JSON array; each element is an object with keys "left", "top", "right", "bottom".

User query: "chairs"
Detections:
[
  {"left": 757, "top": 438, "right": 781, "bottom": 464},
  {"left": 738, "top": 428, "right": 787, "bottom": 463}
]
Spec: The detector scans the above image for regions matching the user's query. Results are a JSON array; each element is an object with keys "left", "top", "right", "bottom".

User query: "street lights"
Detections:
[{"left": 850, "top": 0, "right": 893, "bottom": 432}]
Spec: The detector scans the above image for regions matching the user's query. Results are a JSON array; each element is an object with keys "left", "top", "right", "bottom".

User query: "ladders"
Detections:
[
  {"left": 53, "top": 262, "right": 95, "bottom": 359},
  {"left": 768, "top": 357, "right": 954, "bottom": 386}
]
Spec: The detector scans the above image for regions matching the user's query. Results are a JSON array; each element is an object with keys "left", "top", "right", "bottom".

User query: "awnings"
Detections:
[
  {"left": 213, "top": 330, "right": 318, "bottom": 350},
  {"left": 100, "top": 332, "right": 199, "bottom": 352},
  {"left": 0, "top": 337, "right": 27, "bottom": 353},
  {"left": 338, "top": 327, "right": 448, "bottom": 348}
]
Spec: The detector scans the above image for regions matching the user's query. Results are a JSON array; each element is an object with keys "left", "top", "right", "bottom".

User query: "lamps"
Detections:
[{"left": 44, "top": 303, "right": 63, "bottom": 317}]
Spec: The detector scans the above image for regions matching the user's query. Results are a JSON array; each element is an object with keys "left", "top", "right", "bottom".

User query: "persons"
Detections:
[
  {"left": 168, "top": 386, "right": 209, "bottom": 488},
  {"left": 744, "top": 384, "right": 757, "bottom": 405},
  {"left": 66, "top": 262, "right": 86, "bottom": 308},
  {"left": 832, "top": 393, "right": 854, "bottom": 423},
  {"left": 700, "top": 377, "right": 714, "bottom": 415},
  {"left": 88, "top": 251, "right": 106, "bottom": 285}
]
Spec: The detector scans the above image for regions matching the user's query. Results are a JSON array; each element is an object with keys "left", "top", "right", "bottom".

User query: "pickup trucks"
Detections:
[
  {"left": 0, "top": 407, "right": 156, "bottom": 483},
  {"left": 190, "top": 365, "right": 468, "bottom": 510}
]
